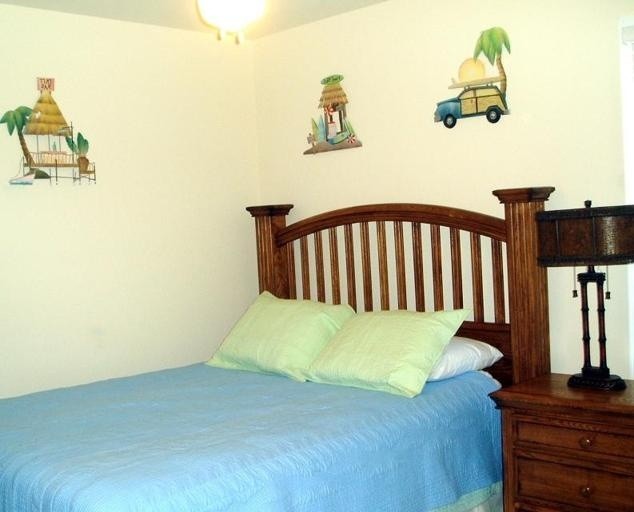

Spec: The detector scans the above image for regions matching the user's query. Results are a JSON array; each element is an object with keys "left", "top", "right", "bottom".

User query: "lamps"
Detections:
[{"left": 530, "top": 196, "right": 634, "bottom": 394}]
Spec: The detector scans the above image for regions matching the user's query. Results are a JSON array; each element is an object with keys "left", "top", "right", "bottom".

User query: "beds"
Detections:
[{"left": 0, "top": 185, "right": 561, "bottom": 511}]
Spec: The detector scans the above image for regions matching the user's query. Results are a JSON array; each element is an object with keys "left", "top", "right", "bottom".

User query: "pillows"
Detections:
[{"left": 205, "top": 288, "right": 504, "bottom": 399}]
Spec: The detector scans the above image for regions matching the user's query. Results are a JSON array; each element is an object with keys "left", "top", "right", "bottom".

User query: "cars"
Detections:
[{"left": 433, "top": 82, "right": 507, "bottom": 128}]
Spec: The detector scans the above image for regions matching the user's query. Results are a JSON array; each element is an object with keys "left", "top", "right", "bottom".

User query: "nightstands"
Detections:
[{"left": 485, "top": 371, "right": 633, "bottom": 512}]
